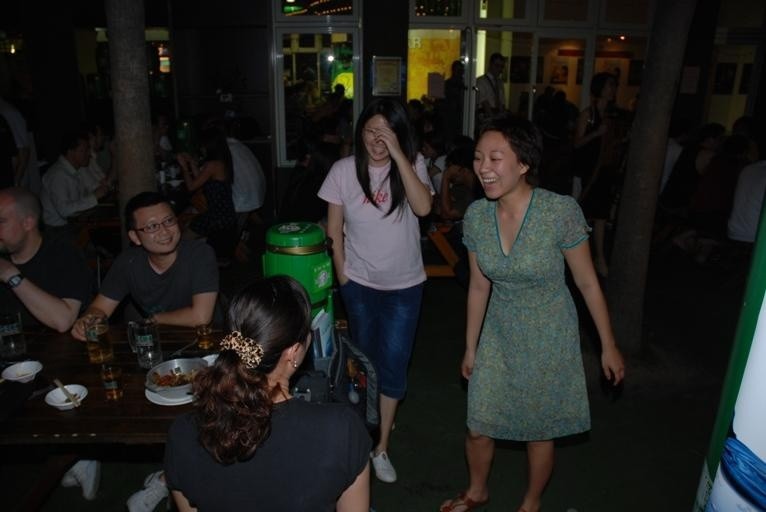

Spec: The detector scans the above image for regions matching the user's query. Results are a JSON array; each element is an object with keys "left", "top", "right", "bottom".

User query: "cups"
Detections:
[
  {"left": 192, "top": 319, "right": 213, "bottom": 350},
  {"left": 126, "top": 315, "right": 161, "bottom": 369},
  {"left": 0, "top": 310, "right": 27, "bottom": 358},
  {"left": 158, "top": 164, "right": 179, "bottom": 185},
  {"left": 96, "top": 358, "right": 124, "bottom": 401},
  {"left": 83, "top": 316, "right": 112, "bottom": 366}
]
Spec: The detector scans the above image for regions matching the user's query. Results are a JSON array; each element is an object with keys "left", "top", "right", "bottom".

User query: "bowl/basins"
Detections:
[
  {"left": 144, "top": 357, "right": 208, "bottom": 400},
  {"left": 43, "top": 384, "right": 87, "bottom": 412},
  {"left": 1, "top": 360, "right": 43, "bottom": 385}
]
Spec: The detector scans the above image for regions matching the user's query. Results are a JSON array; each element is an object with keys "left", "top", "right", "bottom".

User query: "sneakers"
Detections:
[{"left": 369, "top": 451, "right": 396, "bottom": 483}]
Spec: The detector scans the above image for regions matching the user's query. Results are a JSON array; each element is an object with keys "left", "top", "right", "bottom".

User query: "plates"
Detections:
[
  {"left": 201, "top": 353, "right": 218, "bottom": 368},
  {"left": 140, "top": 390, "right": 199, "bottom": 406}
]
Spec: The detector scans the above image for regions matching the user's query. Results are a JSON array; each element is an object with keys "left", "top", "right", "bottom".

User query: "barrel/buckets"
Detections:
[{"left": 262, "top": 222, "right": 336, "bottom": 331}]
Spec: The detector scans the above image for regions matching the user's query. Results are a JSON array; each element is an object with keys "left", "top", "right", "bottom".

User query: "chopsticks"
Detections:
[{"left": 53, "top": 377, "right": 79, "bottom": 408}]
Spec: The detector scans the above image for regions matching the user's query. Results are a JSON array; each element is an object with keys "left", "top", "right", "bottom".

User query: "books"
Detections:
[{"left": 311, "top": 309, "right": 333, "bottom": 358}]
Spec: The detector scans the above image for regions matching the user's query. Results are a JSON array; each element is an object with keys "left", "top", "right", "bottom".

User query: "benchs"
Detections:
[
  {"left": 196, "top": 211, "right": 265, "bottom": 279},
  {"left": 420, "top": 219, "right": 468, "bottom": 279}
]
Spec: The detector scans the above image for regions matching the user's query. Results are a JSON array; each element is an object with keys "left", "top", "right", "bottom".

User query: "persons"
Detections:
[
  {"left": 317, "top": 97, "right": 437, "bottom": 483},
  {"left": 440, "top": 114, "right": 626, "bottom": 512},
  {"left": 70, "top": 192, "right": 224, "bottom": 512},
  {"left": 0, "top": 52, "right": 765, "bottom": 289},
  {"left": 0, "top": 187, "right": 100, "bottom": 488},
  {"left": 164, "top": 275, "right": 373, "bottom": 512}
]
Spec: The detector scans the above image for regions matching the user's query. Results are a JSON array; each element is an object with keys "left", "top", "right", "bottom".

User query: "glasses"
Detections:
[{"left": 134, "top": 217, "right": 178, "bottom": 233}]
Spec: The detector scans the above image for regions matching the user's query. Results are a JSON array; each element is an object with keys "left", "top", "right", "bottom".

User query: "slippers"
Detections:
[{"left": 442, "top": 490, "right": 490, "bottom": 512}]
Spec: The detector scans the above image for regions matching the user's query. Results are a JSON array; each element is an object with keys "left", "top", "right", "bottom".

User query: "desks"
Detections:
[
  {"left": 0, "top": 320, "right": 223, "bottom": 512},
  {"left": 69, "top": 173, "right": 199, "bottom": 227}
]
